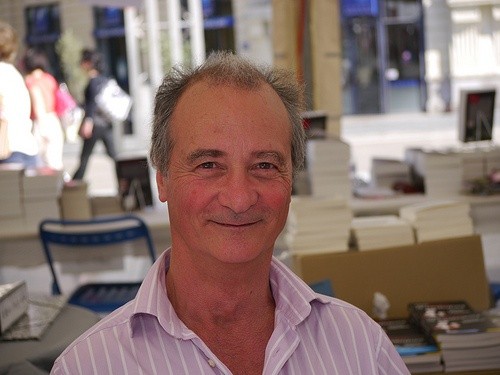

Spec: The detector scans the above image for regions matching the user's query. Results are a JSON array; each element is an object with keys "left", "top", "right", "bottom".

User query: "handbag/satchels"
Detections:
[
  {"left": 94, "top": 78, "right": 132, "bottom": 123},
  {"left": 45, "top": 72, "right": 75, "bottom": 116}
]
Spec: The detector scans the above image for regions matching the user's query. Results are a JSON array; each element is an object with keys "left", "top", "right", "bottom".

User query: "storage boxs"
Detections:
[{"left": 295, "top": 235, "right": 491, "bottom": 321}]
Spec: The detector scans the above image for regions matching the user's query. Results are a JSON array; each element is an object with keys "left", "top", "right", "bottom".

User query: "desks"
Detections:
[{"left": 0, "top": 302, "right": 99, "bottom": 375}]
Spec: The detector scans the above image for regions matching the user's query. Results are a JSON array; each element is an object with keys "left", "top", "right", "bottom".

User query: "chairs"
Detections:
[{"left": 39, "top": 214, "right": 156, "bottom": 313}]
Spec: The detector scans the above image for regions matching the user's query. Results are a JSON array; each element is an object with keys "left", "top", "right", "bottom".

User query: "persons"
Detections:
[
  {"left": 0, "top": 21, "right": 121, "bottom": 180},
  {"left": 49, "top": 49, "right": 411, "bottom": 375}
]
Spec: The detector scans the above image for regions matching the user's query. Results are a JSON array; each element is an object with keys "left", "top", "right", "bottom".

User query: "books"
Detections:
[
  {"left": 0, "top": 162, "right": 122, "bottom": 228},
  {"left": 373, "top": 299, "right": 500, "bottom": 375},
  {"left": 0, "top": 279, "right": 66, "bottom": 343},
  {"left": 274, "top": 140, "right": 500, "bottom": 255}
]
